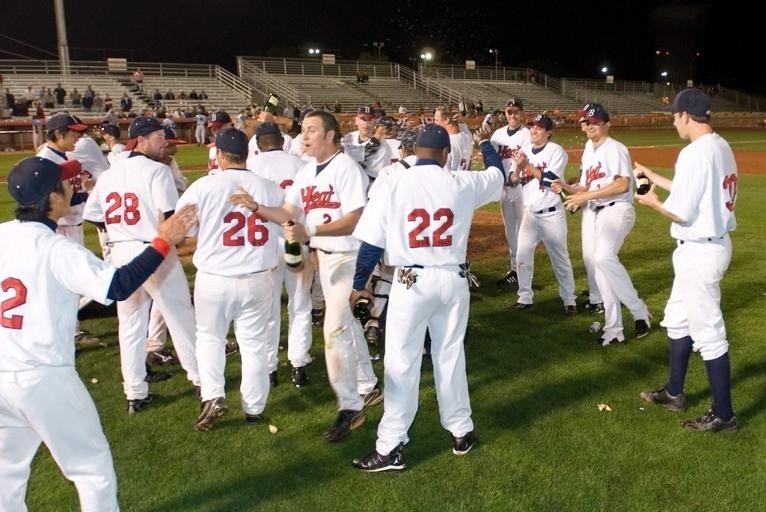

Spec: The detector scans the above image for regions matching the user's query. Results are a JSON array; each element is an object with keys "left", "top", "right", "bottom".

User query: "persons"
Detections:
[
  {"left": 631, "top": 86, "right": 739, "bottom": 436},
  {"left": 0, "top": 156, "right": 201, "bottom": 512}
]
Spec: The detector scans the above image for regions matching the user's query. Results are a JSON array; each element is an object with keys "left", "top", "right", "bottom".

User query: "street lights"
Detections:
[
  {"left": 372, "top": 40, "right": 387, "bottom": 65},
  {"left": 420, "top": 50, "right": 433, "bottom": 66},
  {"left": 489, "top": 48, "right": 499, "bottom": 83}
]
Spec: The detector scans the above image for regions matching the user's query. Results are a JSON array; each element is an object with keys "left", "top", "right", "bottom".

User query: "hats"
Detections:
[
  {"left": 7, "top": 110, "right": 452, "bottom": 212},
  {"left": 504, "top": 88, "right": 711, "bottom": 129}
]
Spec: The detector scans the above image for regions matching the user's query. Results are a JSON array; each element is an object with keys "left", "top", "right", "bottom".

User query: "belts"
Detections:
[
  {"left": 534, "top": 205, "right": 556, "bottom": 216},
  {"left": 592, "top": 199, "right": 616, "bottom": 213}
]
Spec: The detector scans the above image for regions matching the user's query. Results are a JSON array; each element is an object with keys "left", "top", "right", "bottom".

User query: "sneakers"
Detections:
[
  {"left": 503, "top": 268, "right": 739, "bottom": 434},
  {"left": 451, "top": 431, "right": 476, "bottom": 456},
  {"left": 323, "top": 410, "right": 366, "bottom": 441},
  {"left": 269, "top": 370, "right": 279, "bottom": 387},
  {"left": 351, "top": 441, "right": 406, "bottom": 472},
  {"left": 127, "top": 393, "right": 165, "bottom": 414},
  {"left": 145, "top": 371, "right": 174, "bottom": 384},
  {"left": 193, "top": 397, "right": 229, "bottom": 431},
  {"left": 361, "top": 381, "right": 383, "bottom": 408},
  {"left": 224, "top": 342, "right": 240, "bottom": 356},
  {"left": 245, "top": 411, "right": 264, "bottom": 423},
  {"left": 290, "top": 363, "right": 311, "bottom": 387},
  {"left": 147, "top": 349, "right": 179, "bottom": 367}
]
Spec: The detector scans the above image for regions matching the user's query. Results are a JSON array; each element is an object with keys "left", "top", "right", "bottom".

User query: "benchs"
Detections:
[
  {"left": 0, "top": 74, "right": 255, "bottom": 125},
  {"left": 285, "top": 77, "right": 666, "bottom": 115}
]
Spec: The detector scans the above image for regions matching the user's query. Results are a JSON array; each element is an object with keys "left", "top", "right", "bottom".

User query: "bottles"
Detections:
[
  {"left": 265, "top": 94, "right": 280, "bottom": 114},
  {"left": 282, "top": 220, "right": 301, "bottom": 270},
  {"left": 354, "top": 298, "right": 374, "bottom": 323},
  {"left": 558, "top": 185, "right": 582, "bottom": 217},
  {"left": 636, "top": 174, "right": 655, "bottom": 198}
]
